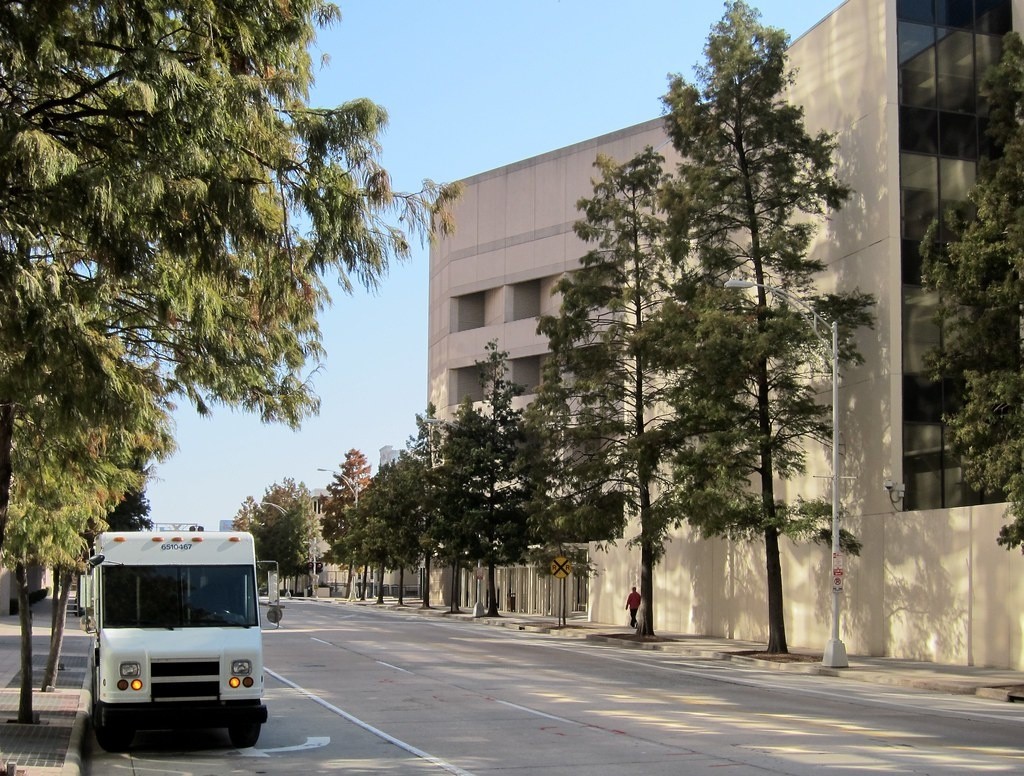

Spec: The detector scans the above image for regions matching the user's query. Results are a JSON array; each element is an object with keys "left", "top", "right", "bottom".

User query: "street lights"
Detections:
[
  {"left": 724, "top": 278, "right": 850, "bottom": 669},
  {"left": 260, "top": 501, "right": 291, "bottom": 597},
  {"left": 423, "top": 418, "right": 485, "bottom": 618},
  {"left": 318, "top": 468, "right": 357, "bottom": 601}
]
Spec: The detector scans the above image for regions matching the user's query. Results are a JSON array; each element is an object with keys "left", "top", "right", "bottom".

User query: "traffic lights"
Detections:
[
  {"left": 316, "top": 563, "right": 323, "bottom": 569},
  {"left": 307, "top": 562, "right": 313, "bottom": 568}
]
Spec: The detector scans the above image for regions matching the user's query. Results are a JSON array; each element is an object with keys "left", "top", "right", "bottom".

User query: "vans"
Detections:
[{"left": 80, "top": 522, "right": 283, "bottom": 751}]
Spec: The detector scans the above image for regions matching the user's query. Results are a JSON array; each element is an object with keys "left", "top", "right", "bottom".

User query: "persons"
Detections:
[{"left": 625, "top": 586, "right": 641, "bottom": 629}]
